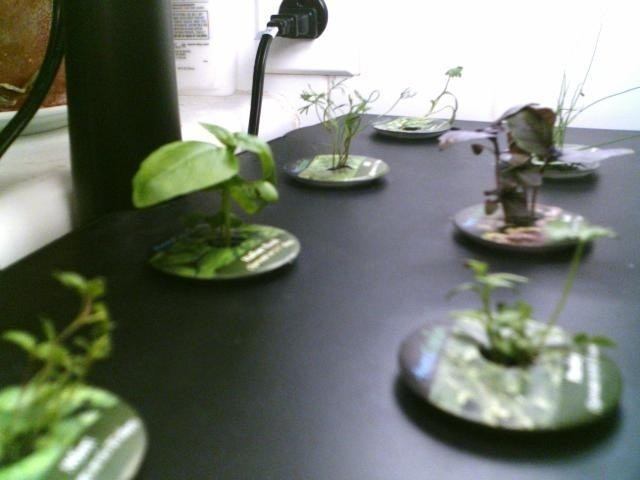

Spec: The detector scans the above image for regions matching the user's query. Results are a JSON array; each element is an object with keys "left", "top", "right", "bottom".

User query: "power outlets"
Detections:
[{"left": 255, "top": 0, "right": 358, "bottom": 75}]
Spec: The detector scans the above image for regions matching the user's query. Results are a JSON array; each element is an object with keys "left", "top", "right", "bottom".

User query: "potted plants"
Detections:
[
  {"left": 132, "top": 122, "right": 298, "bottom": 279},
  {"left": 375, "top": 67, "right": 462, "bottom": 138},
  {"left": 286, "top": 75, "right": 389, "bottom": 185},
  {"left": 496, "top": 30, "right": 639, "bottom": 177},
  {"left": 439, "top": 104, "right": 586, "bottom": 247},
  {"left": 399, "top": 220, "right": 618, "bottom": 431},
  {"left": 0, "top": 272, "right": 148, "bottom": 480}
]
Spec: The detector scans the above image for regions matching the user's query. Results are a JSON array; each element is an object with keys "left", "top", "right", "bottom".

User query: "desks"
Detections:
[{"left": 0, "top": 111, "right": 640, "bottom": 480}]
